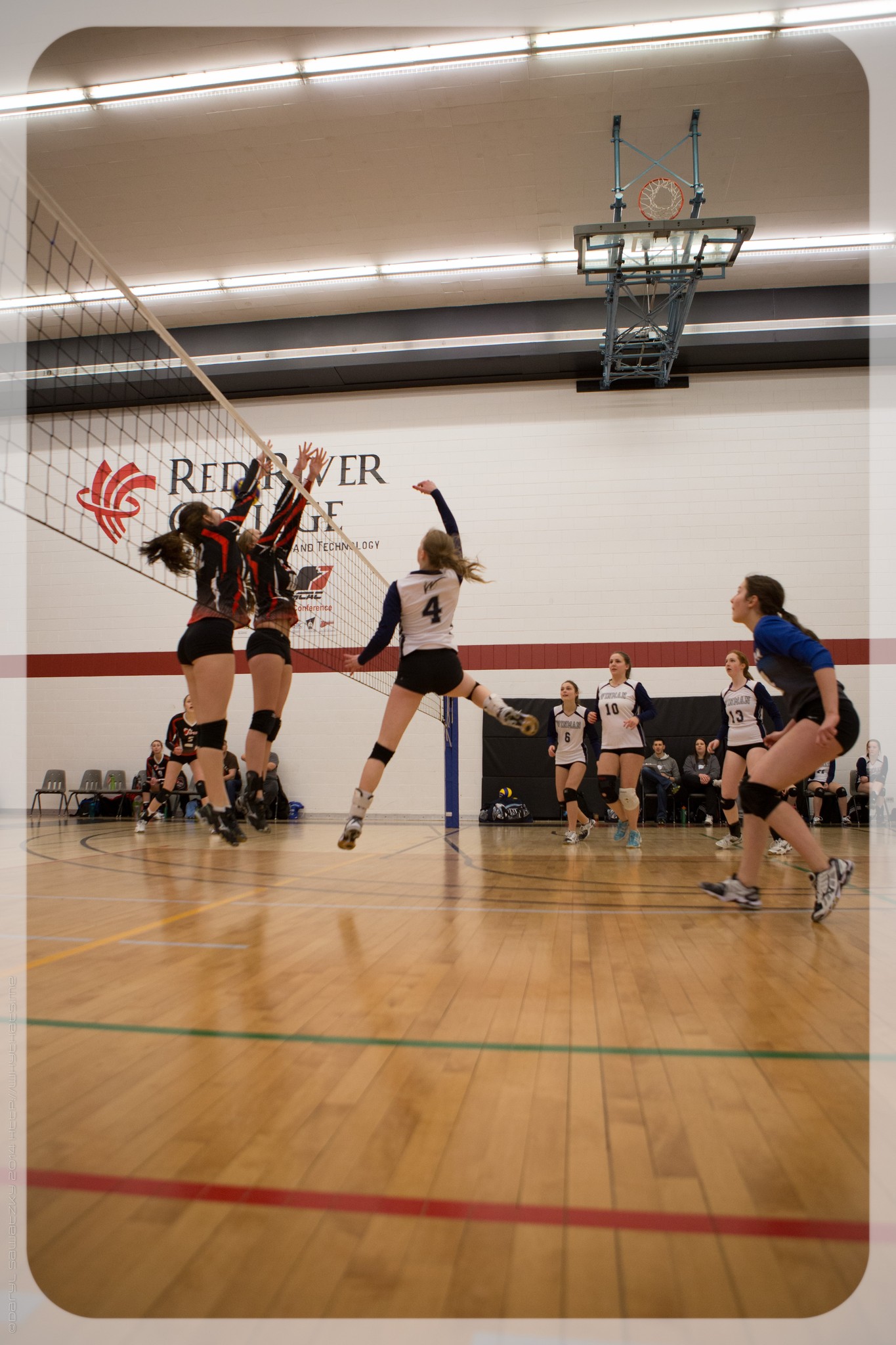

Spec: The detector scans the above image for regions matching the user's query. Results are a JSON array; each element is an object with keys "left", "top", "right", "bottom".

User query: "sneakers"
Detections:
[
  {"left": 614, "top": 819, "right": 642, "bottom": 848},
  {"left": 716, "top": 833, "right": 743, "bottom": 848},
  {"left": 813, "top": 816, "right": 822, "bottom": 825},
  {"left": 841, "top": 815, "right": 852, "bottom": 824},
  {"left": 496, "top": 706, "right": 539, "bottom": 735},
  {"left": 697, "top": 872, "right": 761, "bottom": 909},
  {"left": 235, "top": 791, "right": 271, "bottom": 834},
  {"left": 337, "top": 817, "right": 361, "bottom": 849},
  {"left": 807, "top": 857, "right": 855, "bottom": 921},
  {"left": 767, "top": 838, "right": 793, "bottom": 855},
  {"left": 194, "top": 802, "right": 246, "bottom": 847},
  {"left": 563, "top": 819, "right": 595, "bottom": 845}
]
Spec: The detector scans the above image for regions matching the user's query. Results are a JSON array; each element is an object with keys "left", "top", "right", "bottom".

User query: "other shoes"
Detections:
[
  {"left": 140, "top": 811, "right": 146, "bottom": 816},
  {"left": 865, "top": 799, "right": 876, "bottom": 810},
  {"left": 876, "top": 815, "right": 886, "bottom": 825},
  {"left": 705, "top": 814, "right": 713, "bottom": 825},
  {"left": 658, "top": 819, "right": 665, "bottom": 825},
  {"left": 134, "top": 819, "right": 147, "bottom": 833},
  {"left": 209, "top": 825, "right": 217, "bottom": 834},
  {"left": 153, "top": 812, "right": 164, "bottom": 820},
  {"left": 712, "top": 779, "right": 722, "bottom": 787},
  {"left": 671, "top": 783, "right": 680, "bottom": 794}
]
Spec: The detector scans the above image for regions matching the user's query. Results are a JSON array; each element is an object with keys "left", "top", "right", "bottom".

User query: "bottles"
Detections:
[
  {"left": 137, "top": 775, "right": 142, "bottom": 790},
  {"left": 164, "top": 803, "right": 168, "bottom": 818},
  {"left": 111, "top": 776, "right": 115, "bottom": 790},
  {"left": 681, "top": 805, "right": 687, "bottom": 823}
]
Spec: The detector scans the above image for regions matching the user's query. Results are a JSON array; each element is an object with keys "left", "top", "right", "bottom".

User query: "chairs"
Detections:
[
  {"left": 638, "top": 769, "right": 892, "bottom": 832},
  {"left": 28, "top": 769, "right": 199, "bottom": 819}
]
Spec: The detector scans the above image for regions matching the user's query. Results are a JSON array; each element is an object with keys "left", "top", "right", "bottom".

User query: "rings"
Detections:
[{"left": 629, "top": 724, "right": 631, "bottom": 727}]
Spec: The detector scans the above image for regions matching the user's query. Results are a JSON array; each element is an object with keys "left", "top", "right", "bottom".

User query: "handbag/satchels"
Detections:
[
  {"left": 69, "top": 794, "right": 137, "bottom": 817},
  {"left": 478, "top": 798, "right": 533, "bottom": 823},
  {"left": 289, "top": 801, "right": 304, "bottom": 819}
]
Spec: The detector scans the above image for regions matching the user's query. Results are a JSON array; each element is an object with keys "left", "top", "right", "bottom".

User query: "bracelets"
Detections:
[{"left": 667, "top": 776, "right": 670, "bottom": 779}]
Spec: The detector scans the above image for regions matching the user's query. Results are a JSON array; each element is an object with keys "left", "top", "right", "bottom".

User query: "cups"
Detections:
[{"left": 593, "top": 813, "right": 599, "bottom": 822}]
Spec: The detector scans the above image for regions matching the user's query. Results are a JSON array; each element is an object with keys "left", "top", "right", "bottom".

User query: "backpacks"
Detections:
[{"left": 185, "top": 798, "right": 202, "bottom": 819}]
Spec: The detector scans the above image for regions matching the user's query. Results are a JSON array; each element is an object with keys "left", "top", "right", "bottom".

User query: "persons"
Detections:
[
  {"left": 707, "top": 650, "right": 792, "bottom": 855},
  {"left": 807, "top": 759, "right": 852, "bottom": 825},
  {"left": 134, "top": 694, "right": 218, "bottom": 835},
  {"left": 639, "top": 738, "right": 681, "bottom": 824},
  {"left": 236, "top": 439, "right": 327, "bottom": 836},
  {"left": 700, "top": 581, "right": 861, "bottom": 923},
  {"left": 141, "top": 740, "right": 243, "bottom": 823},
  {"left": 338, "top": 480, "right": 541, "bottom": 850},
  {"left": 782, "top": 785, "right": 798, "bottom": 808},
  {"left": 587, "top": 651, "right": 655, "bottom": 849},
  {"left": 842, "top": 739, "right": 888, "bottom": 828},
  {"left": 139, "top": 436, "right": 274, "bottom": 846},
  {"left": 547, "top": 680, "right": 601, "bottom": 845},
  {"left": 235, "top": 751, "right": 279, "bottom": 834},
  {"left": 682, "top": 737, "right": 722, "bottom": 826}
]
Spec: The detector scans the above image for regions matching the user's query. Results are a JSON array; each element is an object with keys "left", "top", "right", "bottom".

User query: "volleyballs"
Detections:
[
  {"left": 231, "top": 477, "right": 261, "bottom": 506},
  {"left": 498, "top": 787, "right": 513, "bottom": 799}
]
